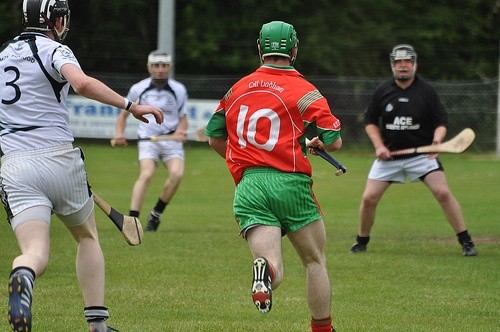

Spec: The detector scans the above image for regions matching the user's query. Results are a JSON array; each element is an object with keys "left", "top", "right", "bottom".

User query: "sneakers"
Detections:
[
  {"left": 251, "top": 257, "right": 272, "bottom": 314},
  {"left": 462, "top": 241, "right": 476, "bottom": 256},
  {"left": 350, "top": 242, "right": 366, "bottom": 252},
  {"left": 90, "top": 322, "right": 120, "bottom": 332},
  {"left": 7, "top": 269, "right": 34, "bottom": 332},
  {"left": 145, "top": 213, "right": 160, "bottom": 231}
]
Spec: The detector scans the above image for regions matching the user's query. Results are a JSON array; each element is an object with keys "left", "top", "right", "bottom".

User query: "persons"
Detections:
[
  {"left": 113, "top": 51, "right": 189, "bottom": 233},
  {"left": 0, "top": 0, "right": 164, "bottom": 332},
  {"left": 204, "top": 20, "right": 343, "bottom": 332},
  {"left": 351, "top": 44, "right": 477, "bottom": 256}
]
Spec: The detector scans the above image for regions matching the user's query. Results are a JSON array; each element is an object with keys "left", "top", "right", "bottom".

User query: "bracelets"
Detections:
[{"left": 123, "top": 99, "right": 133, "bottom": 110}]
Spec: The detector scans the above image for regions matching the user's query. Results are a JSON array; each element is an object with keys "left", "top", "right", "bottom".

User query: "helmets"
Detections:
[
  {"left": 148, "top": 50, "right": 171, "bottom": 89},
  {"left": 390, "top": 44, "right": 417, "bottom": 80},
  {"left": 257, "top": 21, "right": 299, "bottom": 66},
  {"left": 22, "top": 0, "right": 70, "bottom": 43}
]
